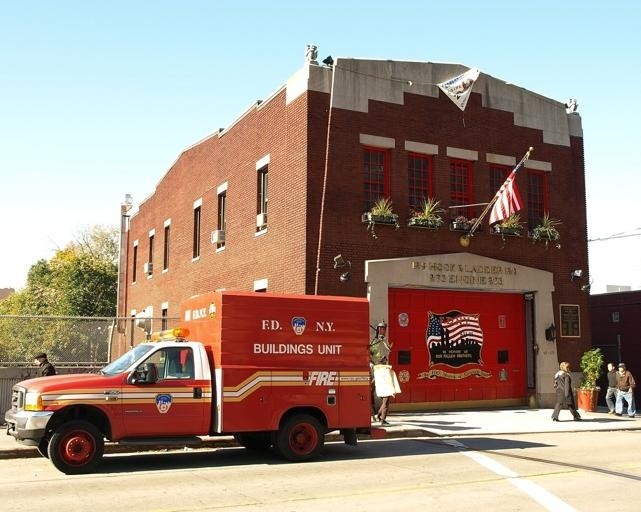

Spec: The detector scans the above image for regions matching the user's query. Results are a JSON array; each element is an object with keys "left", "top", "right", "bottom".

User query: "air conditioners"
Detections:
[
  {"left": 211, "top": 230, "right": 224, "bottom": 244},
  {"left": 144, "top": 263, "right": 153, "bottom": 273},
  {"left": 256, "top": 213, "right": 267, "bottom": 227}
]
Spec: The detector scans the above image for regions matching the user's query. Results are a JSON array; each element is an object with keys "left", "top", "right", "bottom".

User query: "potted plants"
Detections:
[
  {"left": 406, "top": 195, "right": 444, "bottom": 232},
  {"left": 576, "top": 348, "right": 605, "bottom": 412},
  {"left": 492, "top": 214, "right": 528, "bottom": 250},
  {"left": 528, "top": 209, "right": 564, "bottom": 249},
  {"left": 452, "top": 216, "right": 480, "bottom": 230},
  {"left": 361, "top": 196, "right": 400, "bottom": 240}
]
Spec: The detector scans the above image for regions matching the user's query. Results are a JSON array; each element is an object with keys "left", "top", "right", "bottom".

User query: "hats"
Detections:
[
  {"left": 617, "top": 363, "right": 625, "bottom": 367},
  {"left": 33, "top": 353, "right": 45, "bottom": 358}
]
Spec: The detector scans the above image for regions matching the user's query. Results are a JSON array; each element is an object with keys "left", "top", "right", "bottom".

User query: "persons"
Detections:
[
  {"left": 368, "top": 350, "right": 379, "bottom": 420},
  {"left": 550, "top": 359, "right": 583, "bottom": 423},
  {"left": 603, "top": 361, "right": 619, "bottom": 413},
  {"left": 614, "top": 362, "right": 638, "bottom": 419},
  {"left": 372, "top": 354, "right": 402, "bottom": 427},
  {"left": 31, "top": 351, "right": 56, "bottom": 378}
]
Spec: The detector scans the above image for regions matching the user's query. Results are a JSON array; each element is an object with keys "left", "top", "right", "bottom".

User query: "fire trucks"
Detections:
[{"left": 5, "top": 291, "right": 373, "bottom": 474}]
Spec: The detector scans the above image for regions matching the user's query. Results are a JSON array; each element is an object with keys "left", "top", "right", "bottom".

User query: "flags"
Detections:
[
  {"left": 435, "top": 66, "right": 483, "bottom": 113},
  {"left": 487, "top": 162, "right": 525, "bottom": 226}
]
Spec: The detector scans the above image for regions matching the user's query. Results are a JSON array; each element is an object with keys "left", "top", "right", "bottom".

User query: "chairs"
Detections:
[{"left": 167, "top": 352, "right": 183, "bottom": 376}]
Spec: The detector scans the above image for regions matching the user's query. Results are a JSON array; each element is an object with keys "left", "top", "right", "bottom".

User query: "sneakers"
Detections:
[
  {"left": 552, "top": 418, "right": 559, "bottom": 421},
  {"left": 574, "top": 418, "right": 581, "bottom": 421},
  {"left": 377, "top": 414, "right": 390, "bottom": 425},
  {"left": 608, "top": 410, "right": 635, "bottom": 418}
]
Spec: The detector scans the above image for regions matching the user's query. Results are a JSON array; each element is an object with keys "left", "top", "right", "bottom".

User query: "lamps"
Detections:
[{"left": 545, "top": 323, "right": 557, "bottom": 343}]
